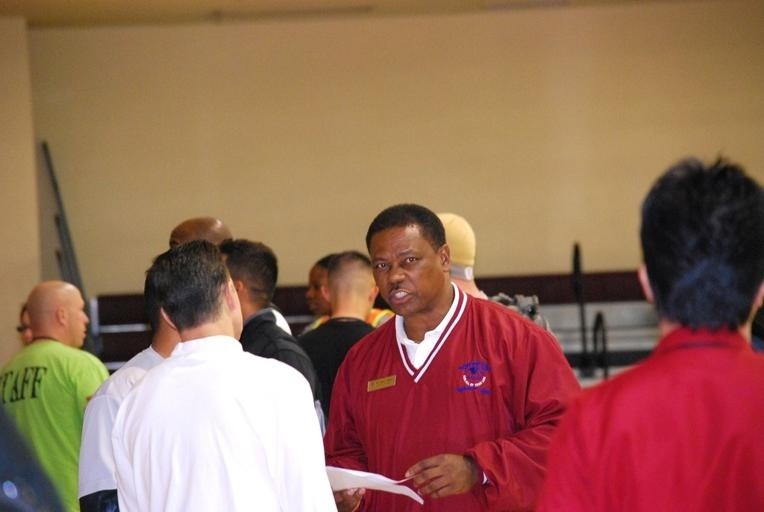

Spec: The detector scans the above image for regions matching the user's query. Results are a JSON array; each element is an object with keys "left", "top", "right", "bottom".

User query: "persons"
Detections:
[
  {"left": 433, "top": 213, "right": 494, "bottom": 303},
  {"left": 75, "top": 250, "right": 182, "bottom": 512},
  {"left": 15, "top": 304, "right": 33, "bottom": 347},
  {"left": 215, "top": 239, "right": 319, "bottom": 405},
  {"left": 0, "top": 275, "right": 111, "bottom": 511},
  {"left": 167, "top": 213, "right": 234, "bottom": 252},
  {"left": 291, "top": 250, "right": 378, "bottom": 422},
  {"left": 321, "top": 202, "right": 586, "bottom": 510},
  {"left": 112, "top": 238, "right": 342, "bottom": 512},
  {"left": 291, "top": 251, "right": 395, "bottom": 341},
  {"left": 535, "top": 145, "right": 763, "bottom": 512}
]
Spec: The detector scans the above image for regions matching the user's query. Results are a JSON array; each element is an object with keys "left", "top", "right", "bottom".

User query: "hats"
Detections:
[{"left": 434, "top": 212, "right": 478, "bottom": 269}]
